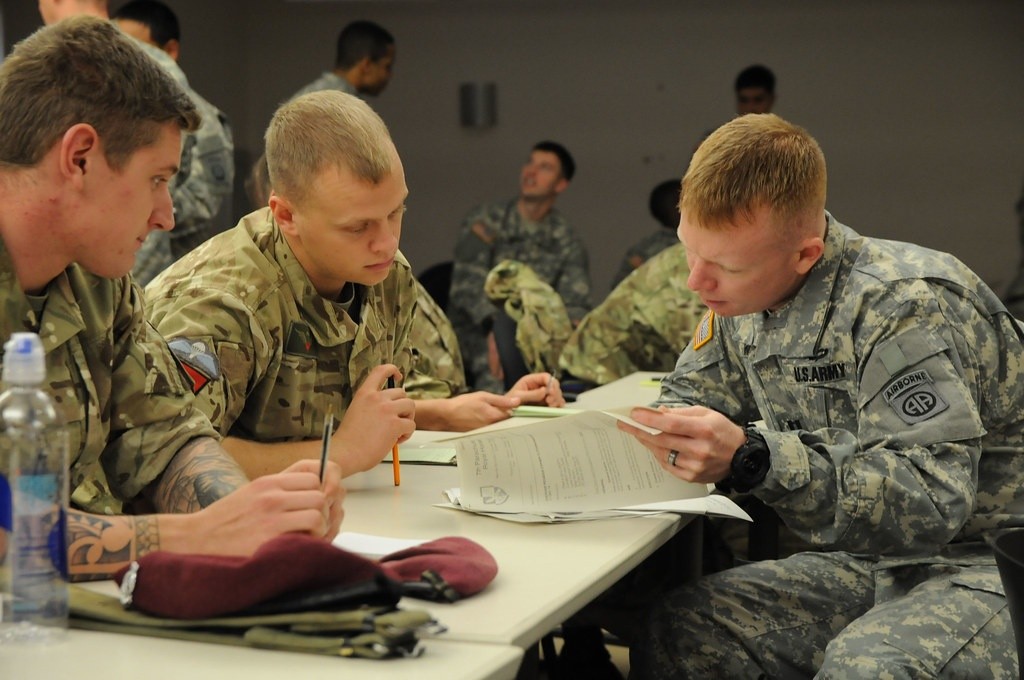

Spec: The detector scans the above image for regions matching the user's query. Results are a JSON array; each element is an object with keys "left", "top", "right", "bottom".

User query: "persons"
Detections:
[
  {"left": 618, "top": 113, "right": 1024, "bottom": 680},
  {"left": 0, "top": 0, "right": 778, "bottom": 583}
]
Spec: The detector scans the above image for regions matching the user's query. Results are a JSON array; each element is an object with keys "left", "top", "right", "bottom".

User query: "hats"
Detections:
[
  {"left": 112, "top": 529, "right": 405, "bottom": 619},
  {"left": 375, "top": 536, "right": 498, "bottom": 602}
]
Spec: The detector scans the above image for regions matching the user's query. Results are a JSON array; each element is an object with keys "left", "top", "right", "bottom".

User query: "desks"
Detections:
[
  {"left": 340, "top": 401, "right": 770, "bottom": 650},
  {"left": 0, "top": 590, "right": 525, "bottom": 680},
  {"left": 576, "top": 371, "right": 769, "bottom": 429}
]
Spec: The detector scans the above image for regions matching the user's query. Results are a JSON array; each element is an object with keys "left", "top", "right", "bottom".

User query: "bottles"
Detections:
[{"left": 0, "top": 333, "right": 68, "bottom": 651}]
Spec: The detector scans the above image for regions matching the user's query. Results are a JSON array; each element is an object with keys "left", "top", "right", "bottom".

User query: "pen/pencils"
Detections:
[
  {"left": 388, "top": 375, "right": 401, "bottom": 486},
  {"left": 319, "top": 403, "right": 334, "bottom": 482},
  {"left": 544, "top": 371, "right": 555, "bottom": 395}
]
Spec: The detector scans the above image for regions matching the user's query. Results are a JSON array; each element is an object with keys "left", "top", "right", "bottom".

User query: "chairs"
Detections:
[
  {"left": 990, "top": 526, "right": 1024, "bottom": 680},
  {"left": 493, "top": 307, "right": 597, "bottom": 399}
]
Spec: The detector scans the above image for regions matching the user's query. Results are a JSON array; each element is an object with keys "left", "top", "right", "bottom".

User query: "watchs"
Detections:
[{"left": 714, "top": 423, "right": 771, "bottom": 495}]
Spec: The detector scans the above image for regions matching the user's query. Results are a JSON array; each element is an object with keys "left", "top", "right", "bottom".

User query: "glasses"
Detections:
[{"left": 736, "top": 96, "right": 769, "bottom": 104}]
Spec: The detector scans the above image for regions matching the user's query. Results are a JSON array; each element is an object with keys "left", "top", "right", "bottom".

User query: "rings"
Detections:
[{"left": 667, "top": 450, "right": 678, "bottom": 466}]
